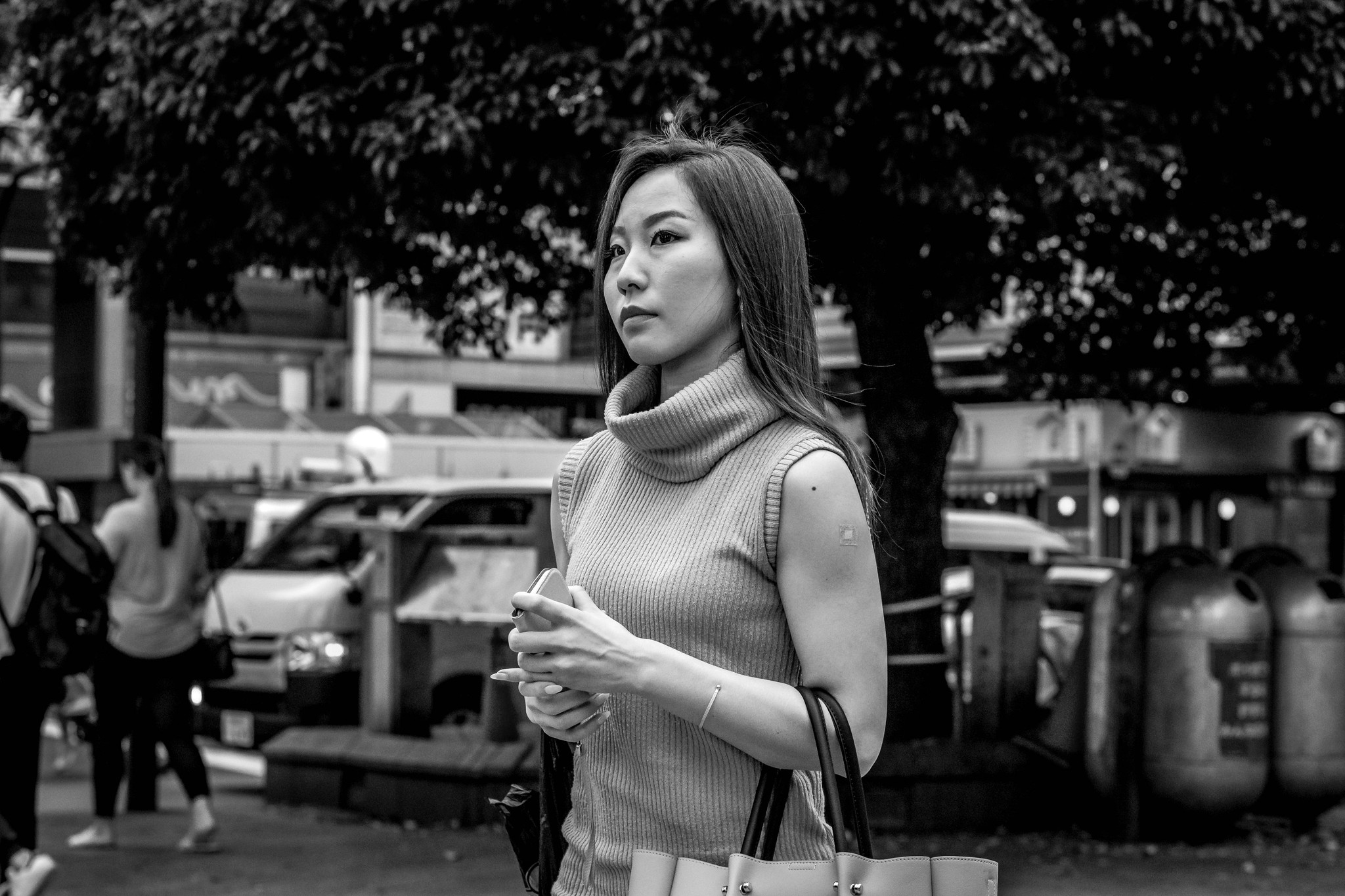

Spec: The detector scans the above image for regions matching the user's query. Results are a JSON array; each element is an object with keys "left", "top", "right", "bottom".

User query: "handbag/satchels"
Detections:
[
  {"left": 628, "top": 685, "right": 998, "bottom": 896},
  {"left": 195, "top": 633, "right": 236, "bottom": 680}
]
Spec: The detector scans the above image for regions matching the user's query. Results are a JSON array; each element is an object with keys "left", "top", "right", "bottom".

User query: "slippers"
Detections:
[
  {"left": 180, "top": 836, "right": 225, "bottom": 853},
  {"left": 68, "top": 828, "right": 116, "bottom": 846}
]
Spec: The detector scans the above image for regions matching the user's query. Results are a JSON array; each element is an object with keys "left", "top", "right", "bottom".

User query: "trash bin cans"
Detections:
[
  {"left": 1146, "top": 564, "right": 1270, "bottom": 816},
  {"left": 1270, "top": 566, "right": 1345, "bottom": 826}
]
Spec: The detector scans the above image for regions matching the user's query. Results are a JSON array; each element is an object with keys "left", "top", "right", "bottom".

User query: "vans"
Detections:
[{"left": 197, "top": 471, "right": 563, "bottom": 755}]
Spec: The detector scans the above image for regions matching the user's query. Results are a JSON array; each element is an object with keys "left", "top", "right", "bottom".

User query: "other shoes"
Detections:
[{"left": 0, "top": 854, "right": 56, "bottom": 896}]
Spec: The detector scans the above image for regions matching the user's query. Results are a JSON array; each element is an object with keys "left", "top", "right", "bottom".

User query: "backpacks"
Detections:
[{"left": 0, "top": 480, "right": 115, "bottom": 677}]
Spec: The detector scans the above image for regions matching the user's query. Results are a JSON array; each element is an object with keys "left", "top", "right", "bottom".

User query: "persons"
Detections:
[
  {"left": 0, "top": 396, "right": 82, "bottom": 896},
  {"left": 489, "top": 134, "right": 888, "bottom": 895},
  {"left": 65, "top": 436, "right": 222, "bottom": 853}
]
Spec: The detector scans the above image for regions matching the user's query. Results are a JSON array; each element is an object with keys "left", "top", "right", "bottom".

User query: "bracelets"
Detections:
[{"left": 699, "top": 684, "right": 721, "bottom": 731}]
